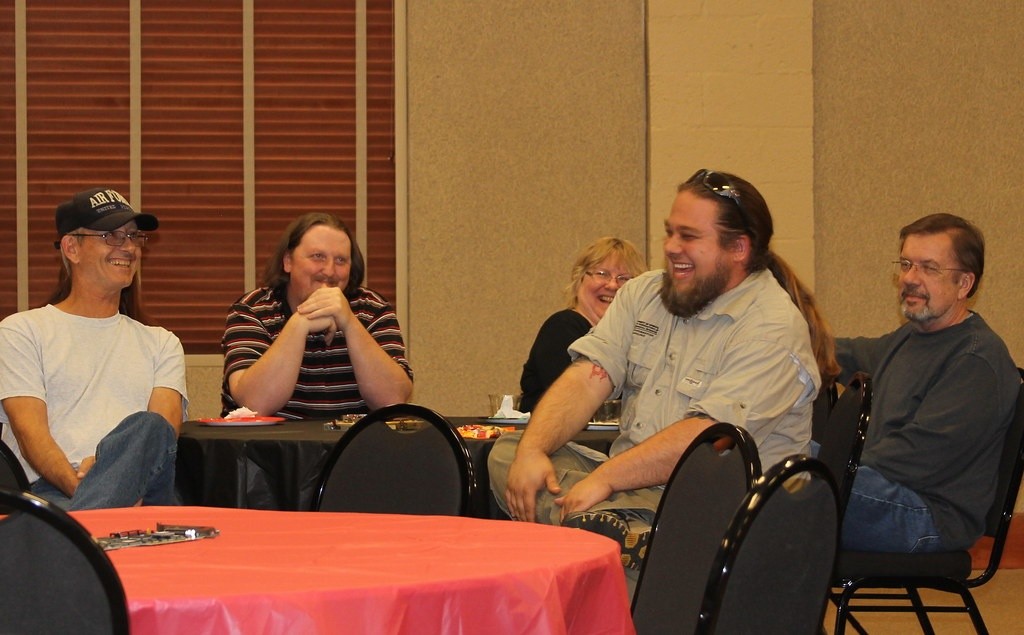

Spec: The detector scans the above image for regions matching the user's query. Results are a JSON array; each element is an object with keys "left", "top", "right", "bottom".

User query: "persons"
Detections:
[
  {"left": 219, "top": 212, "right": 412, "bottom": 508},
  {"left": 834, "top": 214, "right": 1022, "bottom": 553},
  {"left": 519, "top": 236, "right": 646, "bottom": 413},
  {"left": 486, "top": 169, "right": 840, "bottom": 616},
  {"left": 0, "top": 189, "right": 188, "bottom": 512}
]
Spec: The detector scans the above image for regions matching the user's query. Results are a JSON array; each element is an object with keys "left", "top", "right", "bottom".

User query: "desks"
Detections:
[
  {"left": 65, "top": 506, "right": 637, "bottom": 635},
  {"left": 174, "top": 417, "right": 620, "bottom": 520}
]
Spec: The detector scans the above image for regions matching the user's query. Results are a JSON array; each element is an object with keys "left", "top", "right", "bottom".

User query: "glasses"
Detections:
[
  {"left": 68, "top": 230, "right": 150, "bottom": 248},
  {"left": 686, "top": 169, "right": 752, "bottom": 235},
  {"left": 585, "top": 269, "right": 635, "bottom": 288},
  {"left": 890, "top": 260, "right": 969, "bottom": 280}
]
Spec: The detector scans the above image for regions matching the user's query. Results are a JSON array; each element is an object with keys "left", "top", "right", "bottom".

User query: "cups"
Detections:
[
  {"left": 593, "top": 400, "right": 621, "bottom": 424},
  {"left": 489, "top": 394, "right": 518, "bottom": 417}
]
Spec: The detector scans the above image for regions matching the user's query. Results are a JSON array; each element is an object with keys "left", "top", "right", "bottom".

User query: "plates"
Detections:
[
  {"left": 479, "top": 417, "right": 531, "bottom": 424},
  {"left": 584, "top": 424, "right": 619, "bottom": 431},
  {"left": 196, "top": 417, "right": 285, "bottom": 426},
  {"left": 327, "top": 421, "right": 424, "bottom": 430}
]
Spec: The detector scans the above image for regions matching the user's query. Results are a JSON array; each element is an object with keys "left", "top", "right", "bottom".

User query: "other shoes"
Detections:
[{"left": 561, "top": 508, "right": 657, "bottom": 571}]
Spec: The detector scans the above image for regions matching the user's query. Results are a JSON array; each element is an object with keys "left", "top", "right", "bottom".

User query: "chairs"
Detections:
[
  {"left": 309, "top": 403, "right": 477, "bottom": 516},
  {"left": 628, "top": 366, "right": 1024, "bottom": 635},
  {"left": 0, "top": 438, "right": 130, "bottom": 635}
]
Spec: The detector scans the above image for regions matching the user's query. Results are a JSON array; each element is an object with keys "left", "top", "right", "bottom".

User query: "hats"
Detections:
[{"left": 56, "top": 188, "right": 159, "bottom": 236}]
